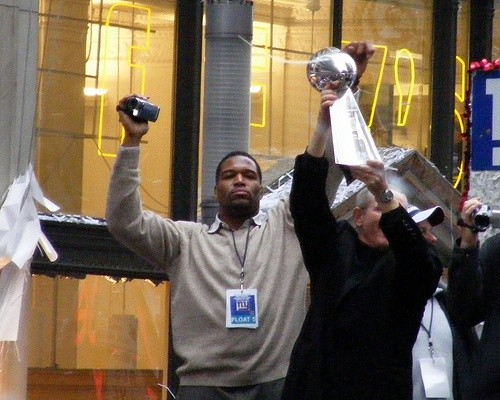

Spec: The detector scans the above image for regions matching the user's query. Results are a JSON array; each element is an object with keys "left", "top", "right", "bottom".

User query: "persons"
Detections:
[
  {"left": 405, "top": 196, "right": 500, "bottom": 400},
  {"left": 282, "top": 80, "right": 444, "bottom": 400},
  {"left": 104, "top": 41, "right": 375, "bottom": 400}
]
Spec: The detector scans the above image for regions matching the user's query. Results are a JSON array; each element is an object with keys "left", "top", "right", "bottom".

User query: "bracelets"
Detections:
[{"left": 354, "top": 78, "right": 361, "bottom": 86}]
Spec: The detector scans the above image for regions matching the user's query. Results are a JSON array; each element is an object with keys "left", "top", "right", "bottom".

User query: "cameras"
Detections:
[
  {"left": 124, "top": 97, "right": 160, "bottom": 123},
  {"left": 472, "top": 204, "right": 500, "bottom": 231}
]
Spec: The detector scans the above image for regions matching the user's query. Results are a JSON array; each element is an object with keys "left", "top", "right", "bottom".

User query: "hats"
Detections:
[{"left": 406, "top": 206, "right": 444, "bottom": 226}]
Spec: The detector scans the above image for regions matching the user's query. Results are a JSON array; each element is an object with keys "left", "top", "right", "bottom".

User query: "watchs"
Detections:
[{"left": 375, "top": 187, "right": 394, "bottom": 202}]
